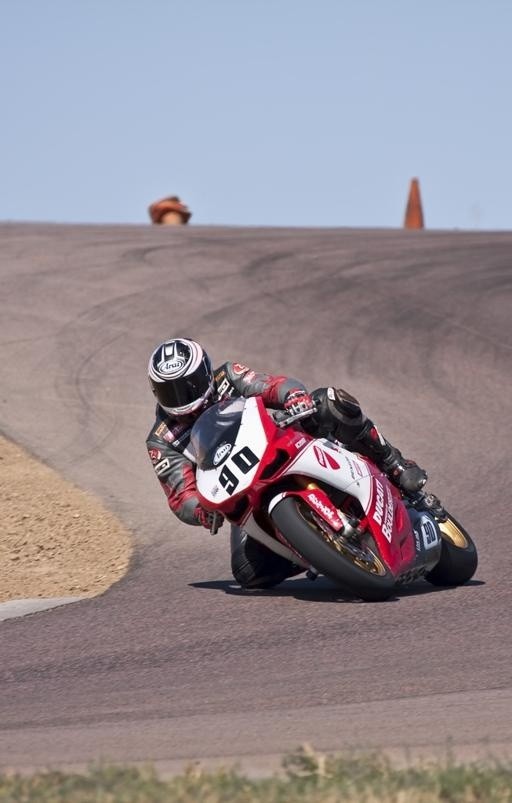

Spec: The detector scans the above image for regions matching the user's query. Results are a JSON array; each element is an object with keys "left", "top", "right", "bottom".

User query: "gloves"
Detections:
[
  {"left": 194, "top": 505, "right": 224, "bottom": 530},
  {"left": 284, "top": 387, "right": 316, "bottom": 416}
]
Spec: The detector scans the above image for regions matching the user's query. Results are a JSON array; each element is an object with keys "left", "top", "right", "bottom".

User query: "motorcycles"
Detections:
[{"left": 191, "top": 395, "right": 478, "bottom": 599}]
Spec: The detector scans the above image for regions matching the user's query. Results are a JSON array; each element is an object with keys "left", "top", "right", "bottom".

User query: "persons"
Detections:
[{"left": 144, "top": 336, "right": 427, "bottom": 589}]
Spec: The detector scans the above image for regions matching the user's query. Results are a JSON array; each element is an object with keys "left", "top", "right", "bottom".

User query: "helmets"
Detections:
[{"left": 149, "top": 339, "right": 215, "bottom": 419}]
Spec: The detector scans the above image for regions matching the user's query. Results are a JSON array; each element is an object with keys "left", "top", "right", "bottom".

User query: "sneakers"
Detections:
[{"left": 382, "top": 447, "right": 427, "bottom": 492}]
